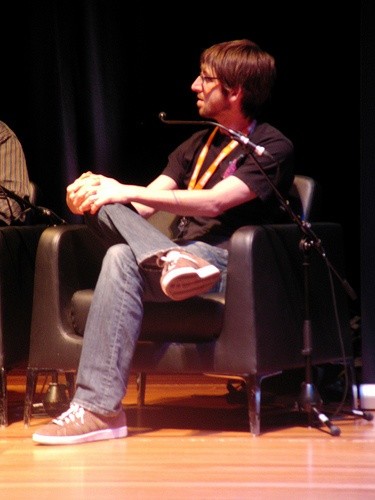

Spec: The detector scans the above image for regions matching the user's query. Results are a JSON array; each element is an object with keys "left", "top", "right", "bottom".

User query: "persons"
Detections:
[
  {"left": 32, "top": 40, "right": 294, "bottom": 445},
  {"left": 0, "top": 121, "right": 35, "bottom": 226}
]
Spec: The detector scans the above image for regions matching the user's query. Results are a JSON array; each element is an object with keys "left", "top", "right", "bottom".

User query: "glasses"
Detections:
[{"left": 198, "top": 72, "right": 219, "bottom": 82}]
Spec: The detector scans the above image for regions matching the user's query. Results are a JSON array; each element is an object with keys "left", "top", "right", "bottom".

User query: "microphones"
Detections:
[{"left": 159, "top": 112, "right": 278, "bottom": 163}]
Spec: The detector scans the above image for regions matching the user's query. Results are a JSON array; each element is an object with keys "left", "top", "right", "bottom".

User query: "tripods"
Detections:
[{"left": 243, "top": 145, "right": 374, "bottom": 435}]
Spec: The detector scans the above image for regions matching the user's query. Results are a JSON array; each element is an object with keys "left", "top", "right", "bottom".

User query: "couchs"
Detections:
[
  {"left": 0, "top": 182, "right": 46, "bottom": 426},
  {"left": 24, "top": 176, "right": 315, "bottom": 439}
]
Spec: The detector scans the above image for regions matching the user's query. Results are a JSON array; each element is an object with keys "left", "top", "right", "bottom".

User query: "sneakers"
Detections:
[
  {"left": 159, "top": 250, "right": 221, "bottom": 299},
  {"left": 32, "top": 401, "right": 127, "bottom": 444}
]
